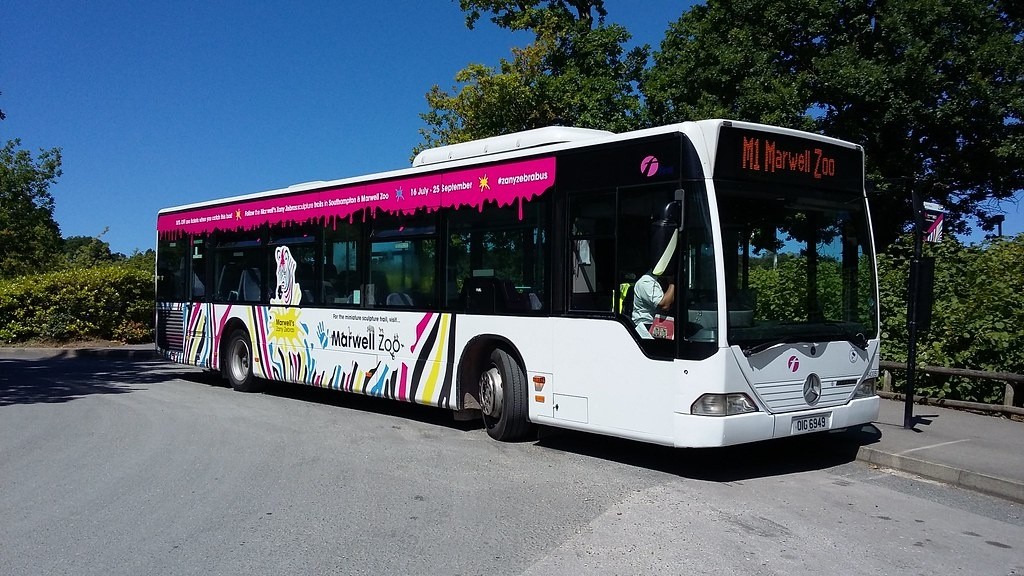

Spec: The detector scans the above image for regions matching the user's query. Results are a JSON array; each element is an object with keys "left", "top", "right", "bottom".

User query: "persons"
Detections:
[{"left": 632, "top": 260, "right": 677, "bottom": 330}]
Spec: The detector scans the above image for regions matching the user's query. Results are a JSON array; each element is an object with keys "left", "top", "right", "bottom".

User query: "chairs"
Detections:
[{"left": 161, "top": 247, "right": 759, "bottom": 325}]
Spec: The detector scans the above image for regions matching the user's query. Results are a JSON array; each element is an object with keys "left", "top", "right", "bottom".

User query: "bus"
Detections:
[{"left": 157, "top": 112, "right": 881, "bottom": 450}]
